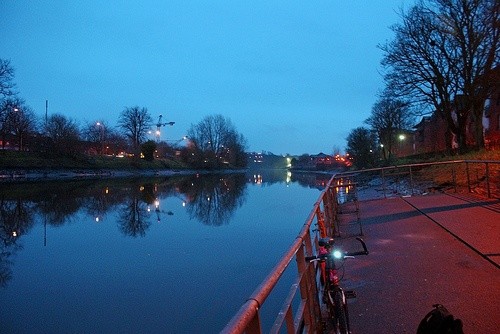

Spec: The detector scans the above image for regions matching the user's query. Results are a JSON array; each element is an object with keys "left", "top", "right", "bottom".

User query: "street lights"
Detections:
[
  {"left": 94, "top": 120, "right": 104, "bottom": 155},
  {"left": 13, "top": 107, "right": 24, "bottom": 155},
  {"left": 10, "top": 191, "right": 23, "bottom": 241},
  {"left": 94, "top": 180, "right": 104, "bottom": 223}
]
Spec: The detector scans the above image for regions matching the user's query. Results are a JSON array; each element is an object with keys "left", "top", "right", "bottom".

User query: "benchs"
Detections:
[{"left": 330, "top": 182, "right": 365, "bottom": 238}]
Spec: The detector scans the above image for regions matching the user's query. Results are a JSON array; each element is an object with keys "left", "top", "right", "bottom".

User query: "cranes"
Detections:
[
  {"left": 144, "top": 114, "right": 188, "bottom": 148},
  {"left": 147, "top": 199, "right": 174, "bottom": 225}
]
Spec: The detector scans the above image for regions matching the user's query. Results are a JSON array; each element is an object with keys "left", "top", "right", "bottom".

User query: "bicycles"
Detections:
[{"left": 304, "top": 232, "right": 369, "bottom": 334}]
